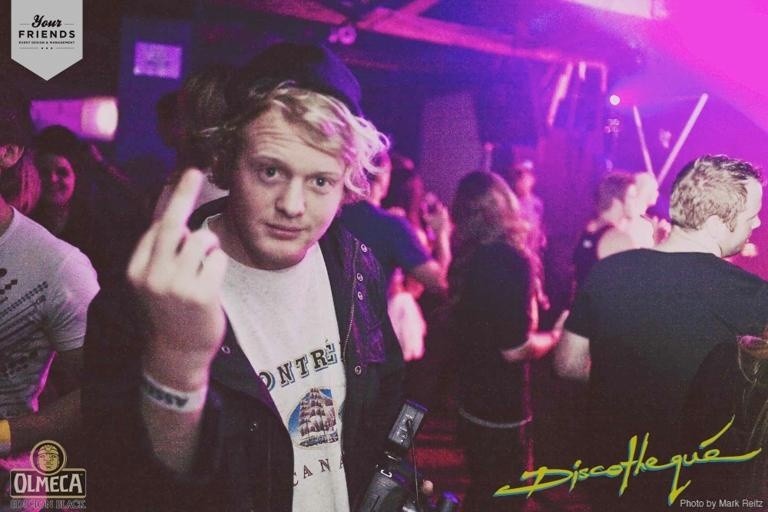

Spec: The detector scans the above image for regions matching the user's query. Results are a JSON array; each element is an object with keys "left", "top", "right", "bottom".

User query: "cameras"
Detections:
[{"left": 353, "top": 398, "right": 463, "bottom": 512}]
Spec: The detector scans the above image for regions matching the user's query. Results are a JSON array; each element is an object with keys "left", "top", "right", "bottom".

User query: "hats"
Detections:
[{"left": 244, "top": 39, "right": 364, "bottom": 119}]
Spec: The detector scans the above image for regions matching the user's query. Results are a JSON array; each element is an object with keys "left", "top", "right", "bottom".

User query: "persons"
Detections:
[{"left": 0, "top": 54, "right": 768, "bottom": 512}]
